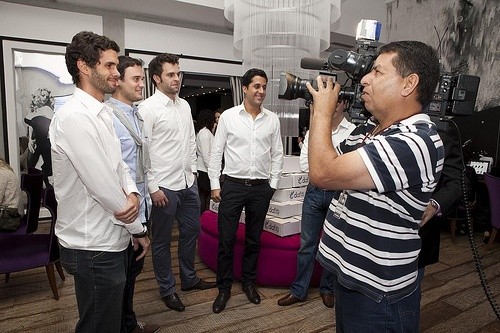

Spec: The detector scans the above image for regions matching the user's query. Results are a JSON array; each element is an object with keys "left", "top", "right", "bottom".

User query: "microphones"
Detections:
[{"left": 301, "top": 57, "right": 327, "bottom": 70}]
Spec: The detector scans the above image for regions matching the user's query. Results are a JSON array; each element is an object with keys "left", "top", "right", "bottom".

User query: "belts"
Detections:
[{"left": 226, "top": 175, "right": 269, "bottom": 187}]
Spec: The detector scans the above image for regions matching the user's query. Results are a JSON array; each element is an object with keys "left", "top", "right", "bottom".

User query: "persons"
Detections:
[
  {"left": 195, "top": 112, "right": 225, "bottom": 215},
  {"left": 278, "top": 99, "right": 356, "bottom": 308},
  {"left": 104, "top": 57, "right": 161, "bottom": 333},
  {"left": 308, "top": 41, "right": 445, "bottom": 333},
  {"left": 138, "top": 54, "right": 217, "bottom": 311},
  {"left": 49, "top": 31, "right": 152, "bottom": 333},
  {"left": 207, "top": 68, "right": 283, "bottom": 313},
  {"left": 420, "top": 129, "right": 482, "bottom": 228},
  {"left": 20, "top": 88, "right": 56, "bottom": 187},
  {"left": 0, "top": 158, "right": 25, "bottom": 219}
]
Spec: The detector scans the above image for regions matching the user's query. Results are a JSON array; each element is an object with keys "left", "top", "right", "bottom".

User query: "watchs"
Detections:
[
  {"left": 430, "top": 201, "right": 438, "bottom": 209},
  {"left": 132, "top": 225, "right": 149, "bottom": 238}
]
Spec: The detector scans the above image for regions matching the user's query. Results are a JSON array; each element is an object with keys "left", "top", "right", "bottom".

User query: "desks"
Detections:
[{"left": 198, "top": 212, "right": 318, "bottom": 289}]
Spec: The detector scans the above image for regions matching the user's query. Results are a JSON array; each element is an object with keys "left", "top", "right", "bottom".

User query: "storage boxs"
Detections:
[{"left": 209, "top": 155, "right": 308, "bottom": 237}]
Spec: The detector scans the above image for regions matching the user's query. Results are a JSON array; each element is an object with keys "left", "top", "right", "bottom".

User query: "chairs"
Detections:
[{"left": 0, "top": 166, "right": 64, "bottom": 301}]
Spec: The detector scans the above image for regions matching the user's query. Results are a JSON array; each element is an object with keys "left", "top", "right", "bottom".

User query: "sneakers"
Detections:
[{"left": 134, "top": 322, "right": 161, "bottom": 333}]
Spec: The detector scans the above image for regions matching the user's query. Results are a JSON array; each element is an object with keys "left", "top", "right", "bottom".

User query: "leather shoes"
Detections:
[
  {"left": 162, "top": 292, "right": 185, "bottom": 312},
  {"left": 278, "top": 294, "right": 307, "bottom": 306},
  {"left": 212, "top": 290, "right": 231, "bottom": 313},
  {"left": 320, "top": 292, "right": 334, "bottom": 308},
  {"left": 181, "top": 279, "right": 217, "bottom": 291},
  {"left": 241, "top": 283, "right": 261, "bottom": 304}
]
